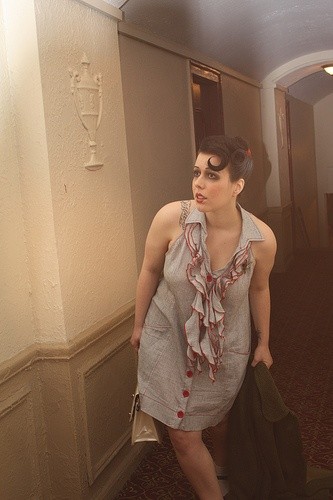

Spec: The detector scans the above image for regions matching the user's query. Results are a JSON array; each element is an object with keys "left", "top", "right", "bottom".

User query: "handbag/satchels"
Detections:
[
  {"left": 238, "top": 361, "right": 312, "bottom": 496},
  {"left": 129, "top": 383, "right": 161, "bottom": 444}
]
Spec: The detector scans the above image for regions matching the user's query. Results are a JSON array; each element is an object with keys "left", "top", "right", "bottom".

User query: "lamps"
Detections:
[{"left": 323, "top": 66, "right": 333, "bottom": 77}]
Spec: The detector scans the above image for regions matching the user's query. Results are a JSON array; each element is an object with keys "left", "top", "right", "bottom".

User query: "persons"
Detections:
[{"left": 129, "top": 135, "right": 277, "bottom": 500}]
